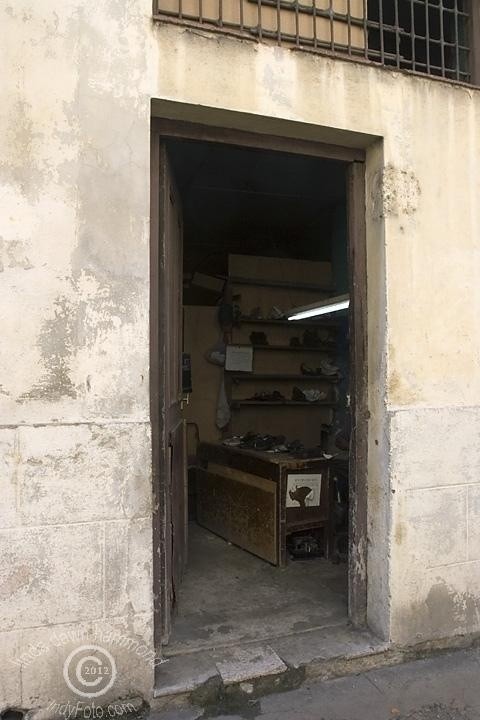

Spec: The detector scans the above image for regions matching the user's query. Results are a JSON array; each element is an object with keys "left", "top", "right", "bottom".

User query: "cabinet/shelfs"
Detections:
[{"left": 225, "top": 276, "right": 339, "bottom": 408}]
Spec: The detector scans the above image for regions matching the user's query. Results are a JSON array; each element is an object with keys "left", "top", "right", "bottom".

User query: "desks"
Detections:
[{"left": 192, "top": 440, "right": 333, "bottom": 567}]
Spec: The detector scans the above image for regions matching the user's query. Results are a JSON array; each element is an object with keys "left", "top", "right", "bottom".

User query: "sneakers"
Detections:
[{"left": 222, "top": 432, "right": 304, "bottom": 453}]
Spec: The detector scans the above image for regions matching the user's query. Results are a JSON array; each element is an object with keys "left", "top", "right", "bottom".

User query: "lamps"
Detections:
[{"left": 286, "top": 292, "right": 351, "bottom": 321}]
